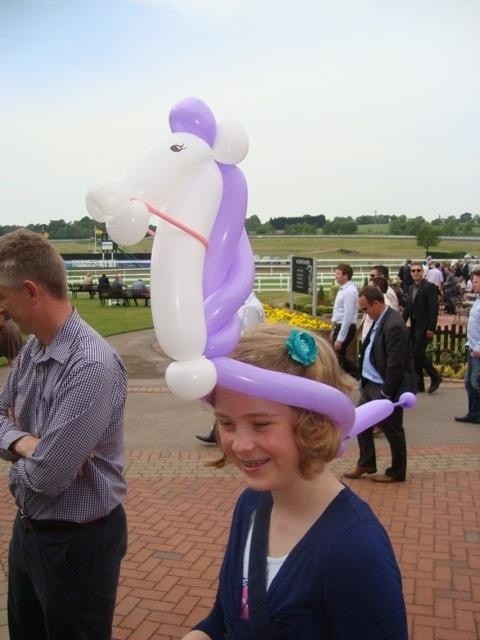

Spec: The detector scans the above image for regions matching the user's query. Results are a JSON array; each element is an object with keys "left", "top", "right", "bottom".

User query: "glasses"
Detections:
[{"left": 412, "top": 270, "right": 420, "bottom": 272}]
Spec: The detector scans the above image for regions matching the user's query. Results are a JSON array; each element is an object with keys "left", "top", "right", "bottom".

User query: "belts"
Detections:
[{"left": 16, "top": 510, "right": 37, "bottom": 531}]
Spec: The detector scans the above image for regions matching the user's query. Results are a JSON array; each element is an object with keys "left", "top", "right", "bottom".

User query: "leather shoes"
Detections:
[
  {"left": 373, "top": 474, "right": 395, "bottom": 482},
  {"left": 196, "top": 434, "right": 215, "bottom": 442},
  {"left": 429, "top": 378, "right": 442, "bottom": 393},
  {"left": 343, "top": 467, "right": 376, "bottom": 478},
  {"left": 455, "top": 415, "right": 472, "bottom": 421}
]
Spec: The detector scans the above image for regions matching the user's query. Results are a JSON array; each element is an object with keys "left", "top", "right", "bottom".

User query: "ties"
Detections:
[{"left": 359, "top": 321, "right": 376, "bottom": 380}]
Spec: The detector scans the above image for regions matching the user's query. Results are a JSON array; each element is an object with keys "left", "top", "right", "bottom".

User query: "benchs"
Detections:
[{"left": 68, "top": 284, "right": 150, "bottom": 307}]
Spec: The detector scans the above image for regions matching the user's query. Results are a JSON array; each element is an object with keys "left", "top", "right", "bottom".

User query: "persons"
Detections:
[
  {"left": 329, "top": 264, "right": 360, "bottom": 381},
  {"left": 194, "top": 416, "right": 218, "bottom": 445},
  {"left": 340, "top": 283, "right": 419, "bottom": 483},
  {"left": 179, "top": 321, "right": 409, "bottom": 639},
  {"left": 0, "top": 229, "right": 130, "bottom": 640},
  {"left": 358, "top": 254, "right": 480, "bottom": 425},
  {"left": 81, "top": 270, "right": 150, "bottom": 307},
  {"left": 0, "top": 312, "right": 25, "bottom": 366}
]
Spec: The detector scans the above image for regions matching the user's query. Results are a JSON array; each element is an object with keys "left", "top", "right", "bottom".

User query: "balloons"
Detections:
[{"left": 86, "top": 95, "right": 417, "bottom": 459}]
